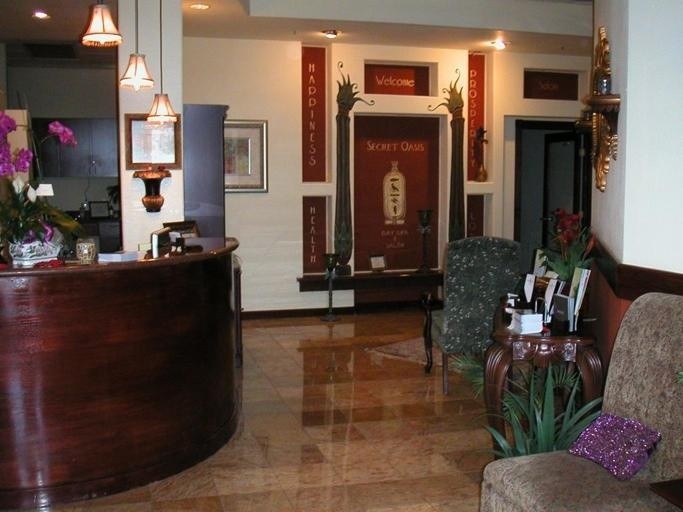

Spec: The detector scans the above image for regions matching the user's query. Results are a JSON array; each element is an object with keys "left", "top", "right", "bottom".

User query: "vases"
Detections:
[
  {"left": 553, "top": 291, "right": 577, "bottom": 322},
  {"left": 9, "top": 232, "right": 63, "bottom": 269},
  {"left": 543, "top": 207, "right": 595, "bottom": 292}
]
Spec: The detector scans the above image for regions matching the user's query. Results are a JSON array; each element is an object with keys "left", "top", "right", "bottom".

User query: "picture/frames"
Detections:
[
  {"left": 224, "top": 119, "right": 268, "bottom": 194},
  {"left": 125, "top": 112, "right": 182, "bottom": 171}
]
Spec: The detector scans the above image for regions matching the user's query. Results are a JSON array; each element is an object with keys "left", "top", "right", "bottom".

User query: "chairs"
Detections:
[{"left": 421, "top": 236, "right": 532, "bottom": 396}]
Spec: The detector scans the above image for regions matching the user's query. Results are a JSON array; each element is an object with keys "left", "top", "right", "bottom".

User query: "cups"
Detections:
[{"left": 76, "top": 243, "right": 95, "bottom": 265}]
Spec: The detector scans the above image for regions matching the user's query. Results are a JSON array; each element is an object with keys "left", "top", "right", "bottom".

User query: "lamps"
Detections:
[
  {"left": 145, "top": 0, "right": 177, "bottom": 129},
  {"left": 120, "top": 0, "right": 153, "bottom": 92},
  {"left": 81, "top": 1, "right": 124, "bottom": 48}
]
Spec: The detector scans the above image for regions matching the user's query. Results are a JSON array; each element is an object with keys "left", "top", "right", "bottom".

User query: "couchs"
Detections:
[{"left": 480, "top": 293, "right": 683, "bottom": 512}]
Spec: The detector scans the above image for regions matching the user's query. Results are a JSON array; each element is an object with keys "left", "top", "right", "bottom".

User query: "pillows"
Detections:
[{"left": 568, "top": 411, "right": 662, "bottom": 480}]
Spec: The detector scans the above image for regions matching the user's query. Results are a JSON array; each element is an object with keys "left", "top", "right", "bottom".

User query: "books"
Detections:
[
  {"left": 96, "top": 250, "right": 138, "bottom": 263},
  {"left": 504, "top": 248, "right": 591, "bottom": 337}
]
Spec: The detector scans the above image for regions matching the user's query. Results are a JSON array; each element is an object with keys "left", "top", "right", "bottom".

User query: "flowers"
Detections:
[{"left": 0, "top": 110, "right": 85, "bottom": 243}]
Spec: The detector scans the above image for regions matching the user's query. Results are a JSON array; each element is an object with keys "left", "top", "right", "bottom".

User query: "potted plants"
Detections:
[{"left": 449, "top": 353, "right": 577, "bottom": 431}]
